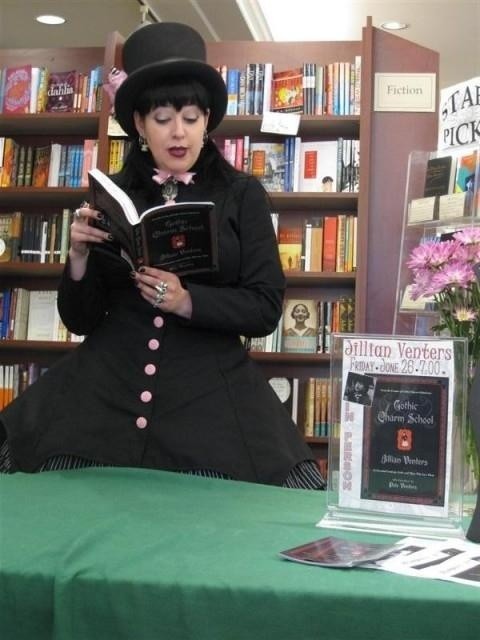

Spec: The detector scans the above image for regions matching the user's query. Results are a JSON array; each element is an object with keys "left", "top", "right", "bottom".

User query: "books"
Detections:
[
  {"left": 209, "top": 56, "right": 360, "bottom": 116},
  {"left": 424, "top": 156, "right": 457, "bottom": 219},
  {"left": 455, "top": 153, "right": 479, "bottom": 218},
  {"left": 271, "top": 213, "right": 357, "bottom": 273},
  {"left": 222, "top": 135, "right": 360, "bottom": 192},
  {"left": 87, "top": 168, "right": 220, "bottom": 281},
  {"left": 281, "top": 536, "right": 410, "bottom": 569},
  {"left": 247, "top": 298, "right": 355, "bottom": 353},
  {"left": 264, "top": 376, "right": 341, "bottom": 439},
  {"left": 0, "top": 65, "right": 100, "bottom": 413}
]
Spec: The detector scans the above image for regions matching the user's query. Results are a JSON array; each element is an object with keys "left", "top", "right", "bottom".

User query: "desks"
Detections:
[{"left": 1, "top": 467, "right": 480, "bottom": 640}]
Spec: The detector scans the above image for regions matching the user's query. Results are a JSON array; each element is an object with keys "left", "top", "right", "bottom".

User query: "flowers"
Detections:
[{"left": 405, "top": 227, "right": 480, "bottom": 495}]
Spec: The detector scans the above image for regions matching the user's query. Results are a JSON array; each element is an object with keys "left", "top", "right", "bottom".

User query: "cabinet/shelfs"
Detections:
[
  {"left": 1, "top": 47, "right": 105, "bottom": 412},
  {"left": 102, "top": 15, "right": 439, "bottom": 492},
  {"left": 392, "top": 144, "right": 480, "bottom": 338}
]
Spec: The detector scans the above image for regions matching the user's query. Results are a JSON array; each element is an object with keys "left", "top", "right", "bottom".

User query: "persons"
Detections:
[
  {"left": 345, "top": 377, "right": 373, "bottom": 407},
  {"left": 0, "top": 22, "right": 327, "bottom": 493}
]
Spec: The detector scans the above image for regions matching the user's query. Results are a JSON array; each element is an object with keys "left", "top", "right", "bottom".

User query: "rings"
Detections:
[
  {"left": 156, "top": 292, "right": 164, "bottom": 300},
  {"left": 153, "top": 285, "right": 168, "bottom": 294},
  {"left": 160, "top": 281, "right": 169, "bottom": 287},
  {"left": 153, "top": 300, "right": 162, "bottom": 308}
]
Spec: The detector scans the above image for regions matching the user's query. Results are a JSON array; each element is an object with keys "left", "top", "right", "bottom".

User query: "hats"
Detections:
[{"left": 114, "top": 21, "right": 228, "bottom": 140}]
